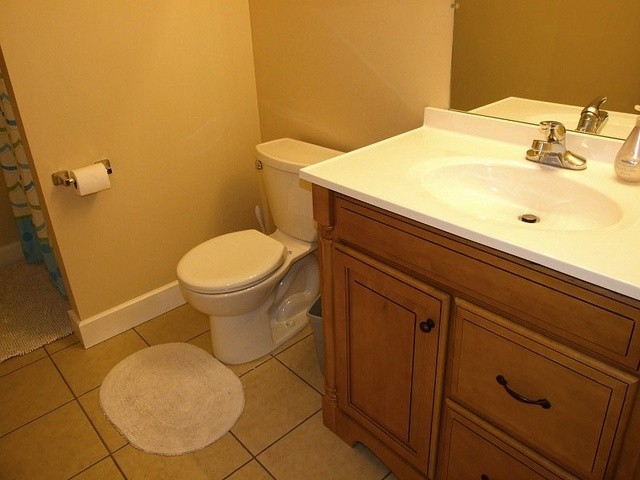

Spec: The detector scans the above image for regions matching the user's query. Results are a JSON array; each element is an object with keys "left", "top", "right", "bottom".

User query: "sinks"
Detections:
[{"left": 407, "top": 157, "right": 630, "bottom": 232}]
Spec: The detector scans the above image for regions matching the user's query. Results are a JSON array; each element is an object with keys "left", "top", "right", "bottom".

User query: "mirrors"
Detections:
[{"left": 449, "top": 0, "right": 640, "bottom": 141}]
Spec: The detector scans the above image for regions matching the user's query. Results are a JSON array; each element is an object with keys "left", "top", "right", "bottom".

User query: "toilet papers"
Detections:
[{"left": 70, "top": 160, "right": 112, "bottom": 197}]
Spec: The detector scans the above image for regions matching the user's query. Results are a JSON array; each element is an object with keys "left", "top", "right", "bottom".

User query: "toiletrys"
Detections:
[{"left": 614, "top": 105, "right": 640, "bottom": 184}]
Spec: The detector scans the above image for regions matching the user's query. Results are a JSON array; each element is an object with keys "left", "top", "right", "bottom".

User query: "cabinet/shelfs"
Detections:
[{"left": 311, "top": 185, "right": 639, "bottom": 480}]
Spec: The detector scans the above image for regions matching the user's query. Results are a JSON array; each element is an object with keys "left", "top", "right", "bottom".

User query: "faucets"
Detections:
[
  {"left": 525, "top": 121, "right": 588, "bottom": 171},
  {"left": 575, "top": 95, "right": 609, "bottom": 135}
]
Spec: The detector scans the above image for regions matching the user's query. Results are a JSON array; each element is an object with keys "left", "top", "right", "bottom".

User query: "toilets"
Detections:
[{"left": 175, "top": 138, "right": 346, "bottom": 367}]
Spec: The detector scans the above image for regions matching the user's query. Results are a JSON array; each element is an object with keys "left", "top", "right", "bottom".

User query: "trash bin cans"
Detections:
[{"left": 306, "top": 296, "right": 324, "bottom": 370}]
